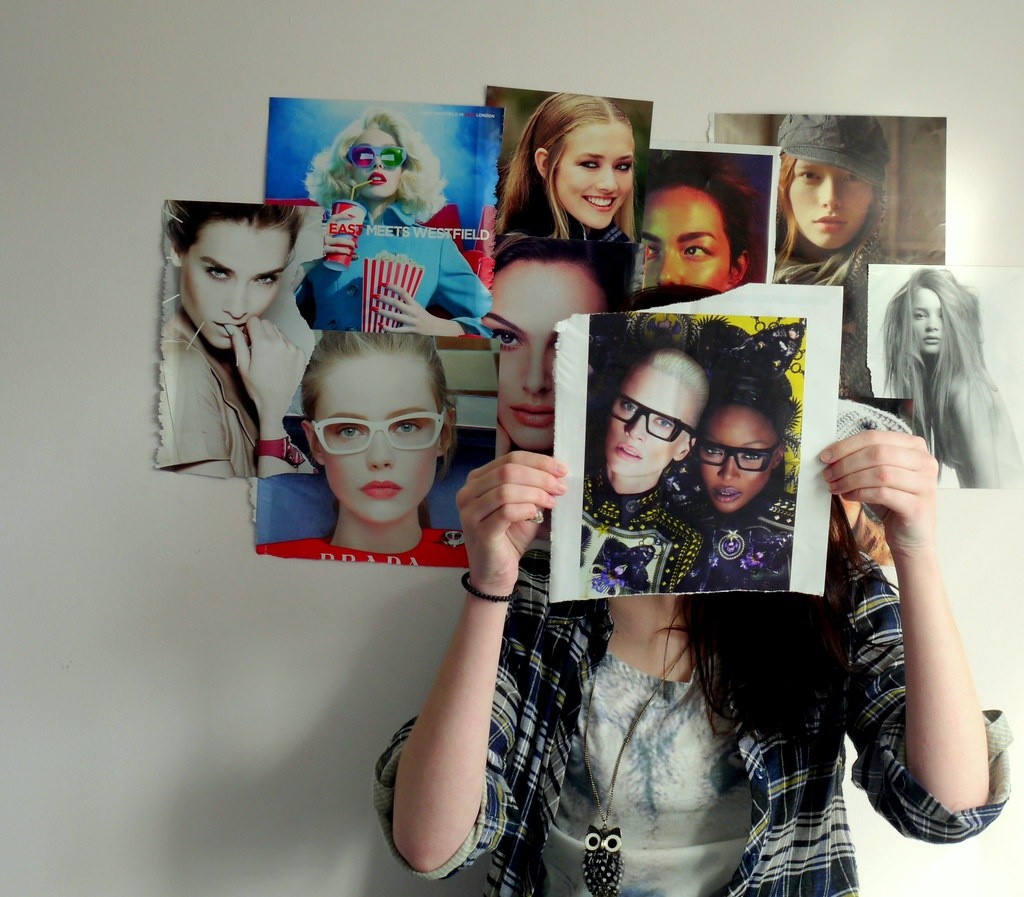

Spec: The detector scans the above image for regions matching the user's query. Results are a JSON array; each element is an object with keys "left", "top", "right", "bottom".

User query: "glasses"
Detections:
[
  {"left": 345, "top": 144, "right": 408, "bottom": 170},
  {"left": 691, "top": 440, "right": 783, "bottom": 472},
  {"left": 608, "top": 391, "right": 697, "bottom": 443},
  {"left": 310, "top": 406, "right": 447, "bottom": 455}
]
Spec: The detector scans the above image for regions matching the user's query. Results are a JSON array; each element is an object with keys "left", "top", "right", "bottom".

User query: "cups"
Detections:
[
  {"left": 323, "top": 200, "right": 367, "bottom": 271},
  {"left": 362, "top": 258, "right": 425, "bottom": 335}
]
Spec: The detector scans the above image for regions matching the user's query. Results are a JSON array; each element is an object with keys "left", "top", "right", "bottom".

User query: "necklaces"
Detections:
[{"left": 581, "top": 645, "right": 688, "bottom": 897}]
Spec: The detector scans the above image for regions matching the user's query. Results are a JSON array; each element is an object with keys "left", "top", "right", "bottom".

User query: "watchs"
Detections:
[{"left": 258, "top": 436, "right": 306, "bottom": 468}]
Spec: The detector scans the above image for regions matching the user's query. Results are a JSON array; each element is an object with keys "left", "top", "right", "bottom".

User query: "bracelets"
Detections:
[{"left": 461, "top": 571, "right": 520, "bottom": 601}]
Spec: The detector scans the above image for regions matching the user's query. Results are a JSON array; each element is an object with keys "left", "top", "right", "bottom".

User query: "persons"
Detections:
[
  {"left": 255, "top": 332, "right": 471, "bottom": 568},
  {"left": 579, "top": 348, "right": 797, "bottom": 595},
  {"left": 291, "top": 108, "right": 495, "bottom": 338},
  {"left": 839, "top": 491, "right": 895, "bottom": 565},
  {"left": 480, "top": 237, "right": 631, "bottom": 459},
  {"left": 882, "top": 267, "right": 1024, "bottom": 488},
  {"left": 157, "top": 199, "right": 308, "bottom": 478},
  {"left": 495, "top": 92, "right": 639, "bottom": 244},
  {"left": 372, "top": 430, "right": 1012, "bottom": 897},
  {"left": 633, "top": 165, "right": 751, "bottom": 293},
  {"left": 773, "top": 114, "right": 899, "bottom": 399}
]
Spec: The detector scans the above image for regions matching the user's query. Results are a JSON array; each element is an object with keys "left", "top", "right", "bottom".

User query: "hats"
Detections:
[{"left": 777, "top": 115, "right": 890, "bottom": 187}]
